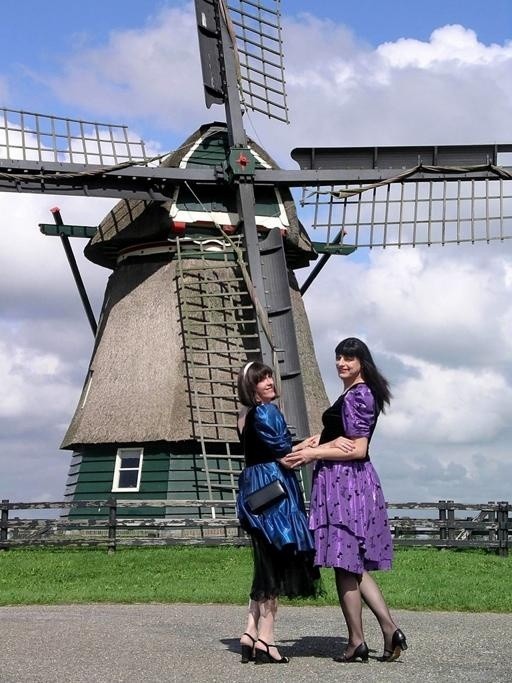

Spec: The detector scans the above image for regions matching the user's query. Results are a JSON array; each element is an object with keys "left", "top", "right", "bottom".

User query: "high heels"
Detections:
[
  {"left": 333, "top": 641, "right": 368, "bottom": 663},
  {"left": 377, "top": 629, "right": 408, "bottom": 662},
  {"left": 255, "top": 639, "right": 289, "bottom": 664},
  {"left": 241, "top": 633, "right": 257, "bottom": 663}
]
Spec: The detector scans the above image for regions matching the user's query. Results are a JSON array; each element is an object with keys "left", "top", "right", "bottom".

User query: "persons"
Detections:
[
  {"left": 235, "top": 359, "right": 356, "bottom": 664},
  {"left": 284, "top": 338, "right": 408, "bottom": 663}
]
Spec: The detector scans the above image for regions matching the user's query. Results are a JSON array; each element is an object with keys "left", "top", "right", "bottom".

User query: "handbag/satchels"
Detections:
[{"left": 244, "top": 479, "right": 287, "bottom": 515}]
